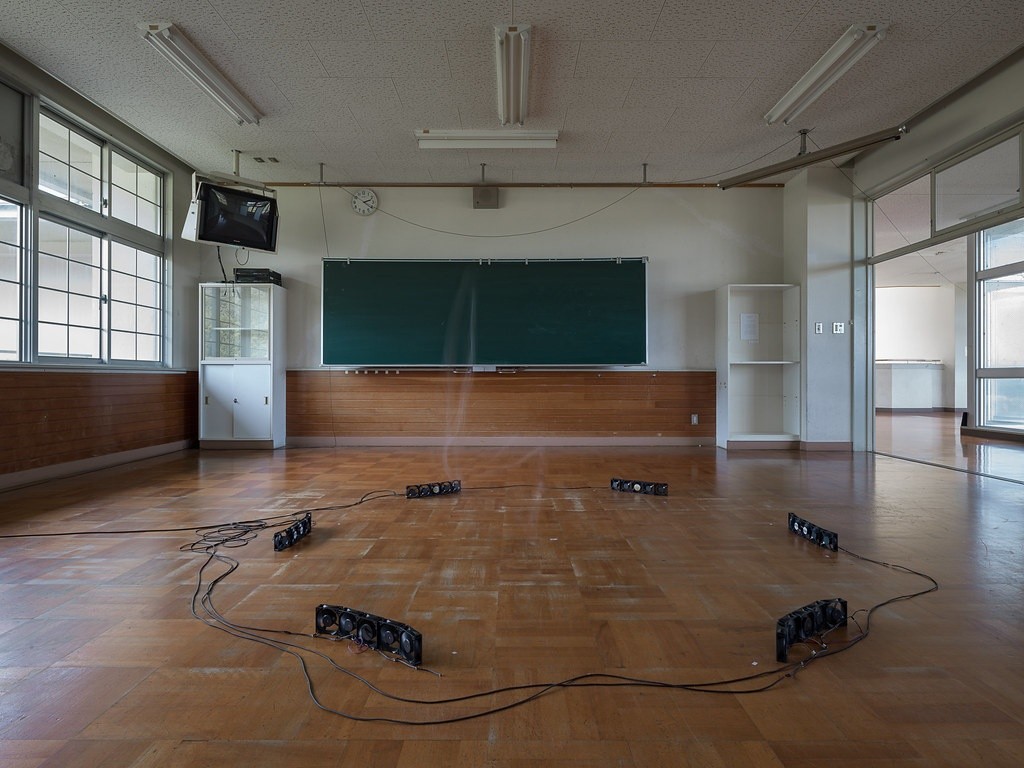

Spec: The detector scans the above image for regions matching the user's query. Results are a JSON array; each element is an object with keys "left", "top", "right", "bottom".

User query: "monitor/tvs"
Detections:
[{"left": 195, "top": 181, "right": 278, "bottom": 252}]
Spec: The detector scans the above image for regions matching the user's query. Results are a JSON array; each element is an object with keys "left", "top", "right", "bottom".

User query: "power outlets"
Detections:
[{"left": 691, "top": 414, "right": 699, "bottom": 425}]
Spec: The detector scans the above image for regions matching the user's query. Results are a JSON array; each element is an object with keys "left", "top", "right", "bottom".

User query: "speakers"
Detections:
[{"left": 472, "top": 187, "right": 499, "bottom": 209}]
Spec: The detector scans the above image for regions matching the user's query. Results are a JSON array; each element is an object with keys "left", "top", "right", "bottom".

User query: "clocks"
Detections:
[{"left": 352, "top": 190, "right": 378, "bottom": 216}]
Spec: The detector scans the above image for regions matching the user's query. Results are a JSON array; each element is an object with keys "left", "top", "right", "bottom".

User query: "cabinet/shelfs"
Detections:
[
  {"left": 715, "top": 283, "right": 801, "bottom": 450},
  {"left": 199, "top": 283, "right": 285, "bottom": 450}
]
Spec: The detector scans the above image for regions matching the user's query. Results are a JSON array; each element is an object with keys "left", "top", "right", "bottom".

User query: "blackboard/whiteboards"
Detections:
[{"left": 320, "top": 256, "right": 650, "bottom": 371}]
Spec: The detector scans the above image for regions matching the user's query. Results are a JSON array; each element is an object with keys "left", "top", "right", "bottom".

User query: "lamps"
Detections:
[
  {"left": 141, "top": 24, "right": 263, "bottom": 126},
  {"left": 764, "top": 23, "right": 888, "bottom": 127},
  {"left": 495, "top": 24, "right": 532, "bottom": 126}
]
